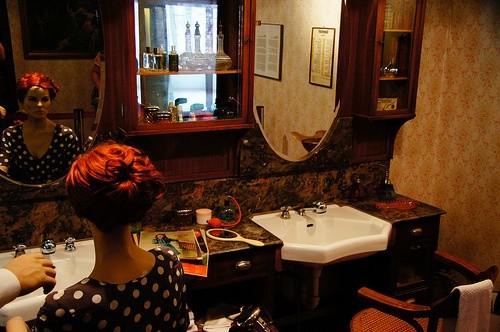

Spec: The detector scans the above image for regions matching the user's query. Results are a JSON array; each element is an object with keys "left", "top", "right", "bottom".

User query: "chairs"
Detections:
[{"left": 350, "top": 250, "right": 499, "bottom": 332}]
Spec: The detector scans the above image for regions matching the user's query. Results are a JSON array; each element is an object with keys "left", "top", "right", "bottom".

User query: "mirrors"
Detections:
[
  {"left": 0, "top": 0, "right": 106, "bottom": 190},
  {"left": 255, "top": 0, "right": 354, "bottom": 163}
]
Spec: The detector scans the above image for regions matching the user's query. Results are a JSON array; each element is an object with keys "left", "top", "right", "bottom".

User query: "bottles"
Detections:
[
  {"left": 216, "top": 21, "right": 232, "bottom": 70},
  {"left": 384, "top": 58, "right": 399, "bottom": 78},
  {"left": 187, "top": 22, "right": 210, "bottom": 71},
  {"left": 178, "top": 23, "right": 195, "bottom": 72},
  {"left": 202, "top": 22, "right": 216, "bottom": 70}
]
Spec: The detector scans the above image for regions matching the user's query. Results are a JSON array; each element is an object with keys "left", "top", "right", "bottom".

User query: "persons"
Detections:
[
  {"left": 91, "top": 50, "right": 101, "bottom": 96},
  {"left": 0, "top": 252, "right": 57, "bottom": 309},
  {"left": 32, "top": 144, "right": 190, "bottom": 332},
  {"left": 0, "top": 72, "right": 83, "bottom": 185},
  {"left": 0, "top": 106, "right": 6, "bottom": 119}
]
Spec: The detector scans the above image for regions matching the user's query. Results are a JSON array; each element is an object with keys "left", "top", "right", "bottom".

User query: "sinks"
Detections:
[
  {"left": 251, "top": 204, "right": 392, "bottom": 263},
  {"left": 0, "top": 238, "right": 96, "bottom": 326}
]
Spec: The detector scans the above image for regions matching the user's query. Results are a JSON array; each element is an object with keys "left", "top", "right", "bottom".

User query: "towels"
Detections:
[{"left": 440, "top": 279, "right": 494, "bottom": 332}]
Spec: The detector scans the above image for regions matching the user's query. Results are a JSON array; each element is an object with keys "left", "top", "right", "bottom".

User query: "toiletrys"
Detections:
[
  {"left": 348, "top": 171, "right": 367, "bottom": 203},
  {"left": 377, "top": 164, "right": 395, "bottom": 202}
]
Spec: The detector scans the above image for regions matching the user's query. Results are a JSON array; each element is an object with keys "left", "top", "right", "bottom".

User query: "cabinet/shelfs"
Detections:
[
  {"left": 107, "top": 0, "right": 255, "bottom": 137},
  {"left": 357, "top": 194, "right": 447, "bottom": 299},
  {"left": 356, "top": 0, "right": 426, "bottom": 120},
  {"left": 141, "top": 216, "right": 284, "bottom": 319}
]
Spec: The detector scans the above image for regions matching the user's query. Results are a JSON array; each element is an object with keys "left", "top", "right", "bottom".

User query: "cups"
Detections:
[{"left": 196, "top": 208, "right": 212, "bottom": 225}]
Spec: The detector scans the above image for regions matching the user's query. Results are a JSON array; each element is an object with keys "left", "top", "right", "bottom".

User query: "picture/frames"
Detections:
[{"left": 18, "top": 0, "right": 106, "bottom": 60}]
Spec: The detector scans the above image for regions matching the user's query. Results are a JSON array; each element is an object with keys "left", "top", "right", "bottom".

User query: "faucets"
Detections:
[
  {"left": 293, "top": 204, "right": 327, "bottom": 216},
  {"left": 41, "top": 239, "right": 56, "bottom": 255}
]
